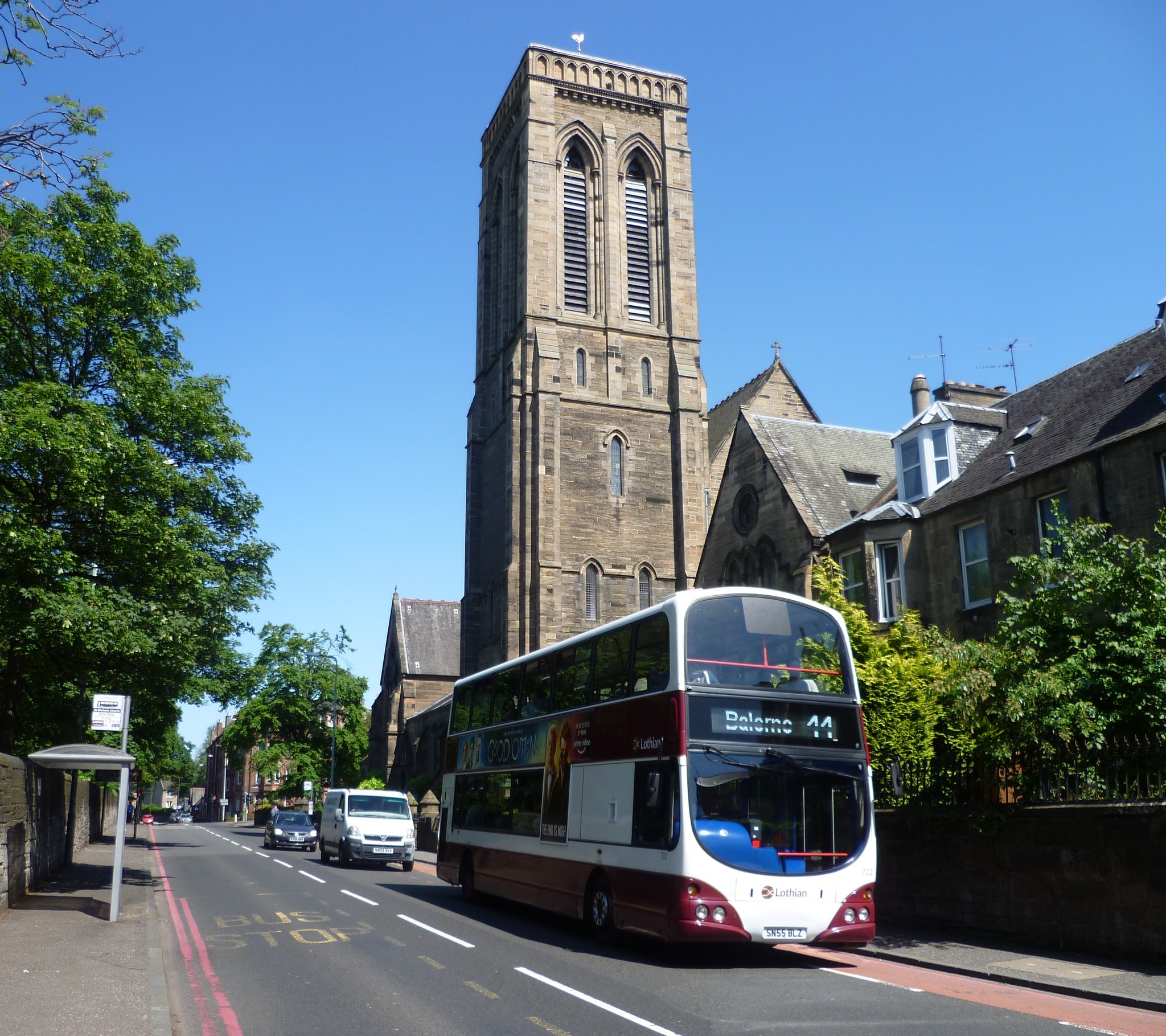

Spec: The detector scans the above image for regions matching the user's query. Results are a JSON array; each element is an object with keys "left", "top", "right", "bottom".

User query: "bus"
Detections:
[{"left": 435, "top": 584, "right": 905, "bottom": 947}]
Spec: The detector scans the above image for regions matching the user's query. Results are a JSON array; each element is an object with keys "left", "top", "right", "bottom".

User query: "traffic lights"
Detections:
[
  {"left": 245, "top": 792, "right": 250, "bottom": 803},
  {"left": 242, "top": 792, "right": 246, "bottom": 803},
  {"left": 249, "top": 794, "right": 254, "bottom": 805},
  {"left": 213, "top": 794, "right": 217, "bottom": 802}
]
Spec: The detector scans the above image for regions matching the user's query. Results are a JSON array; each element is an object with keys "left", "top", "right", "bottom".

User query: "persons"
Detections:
[
  {"left": 522, "top": 691, "right": 550, "bottom": 717},
  {"left": 458, "top": 734, "right": 483, "bottom": 771},
  {"left": 686, "top": 641, "right": 720, "bottom": 684},
  {"left": 758, "top": 657, "right": 820, "bottom": 693},
  {"left": 271, "top": 805, "right": 279, "bottom": 818},
  {"left": 350, "top": 801, "right": 360, "bottom": 812},
  {"left": 544, "top": 721, "right": 570, "bottom": 827}
]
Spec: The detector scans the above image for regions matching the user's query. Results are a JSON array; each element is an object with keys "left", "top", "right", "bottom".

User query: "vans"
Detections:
[{"left": 318, "top": 788, "right": 421, "bottom": 871}]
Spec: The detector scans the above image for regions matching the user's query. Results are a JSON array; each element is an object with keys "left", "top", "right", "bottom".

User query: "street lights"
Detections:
[
  {"left": 199, "top": 764, "right": 211, "bottom": 821},
  {"left": 303, "top": 652, "right": 338, "bottom": 790},
  {"left": 177, "top": 777, "right": 183, "bottom": 813},
  {"left": 207, "top": 754, "right": 223, "bottom": 822}
]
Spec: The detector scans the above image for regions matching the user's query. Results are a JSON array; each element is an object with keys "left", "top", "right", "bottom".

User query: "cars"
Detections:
[
  {"left": 169, "top": 811, "right": 194, "bottom": 824},
  {"left": 142, "top": 814, "right": 154, "bottom": 826},
  {"left": 263, "top": 811, "right": 319, "bottom": 852}
]
